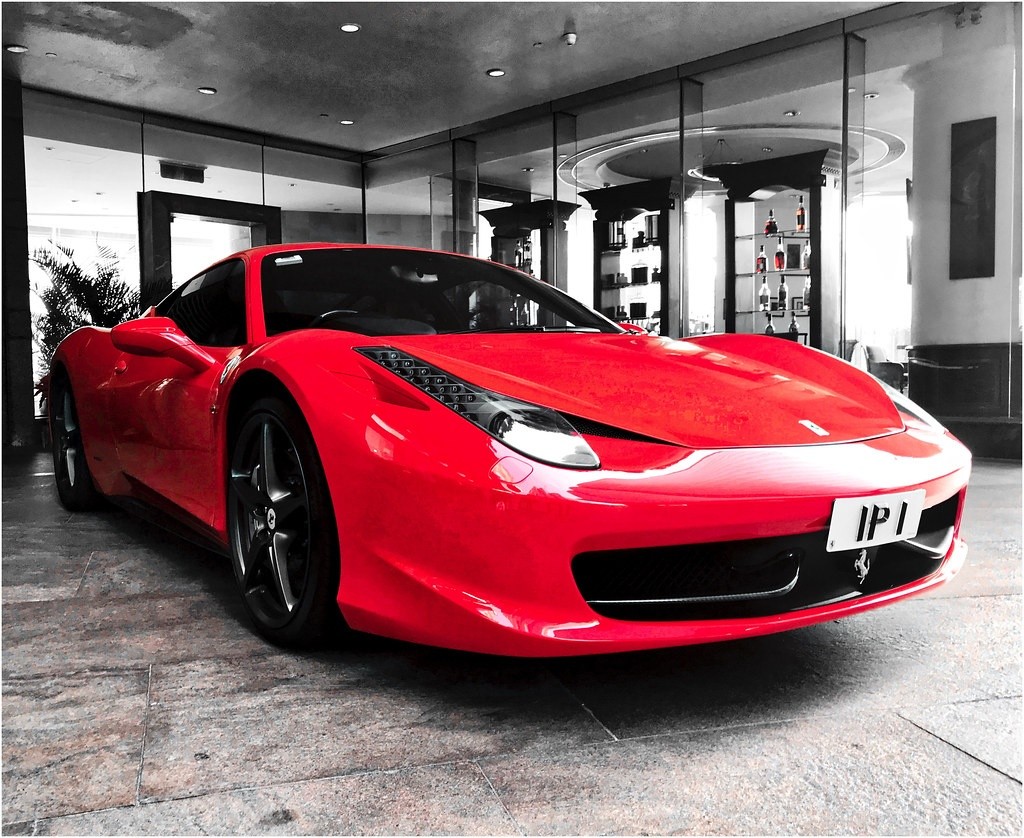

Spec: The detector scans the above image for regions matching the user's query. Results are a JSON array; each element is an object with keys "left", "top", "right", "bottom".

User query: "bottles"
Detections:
[
  {"left": 510, "top": 301, "right": 518, "bottom": 327},
  {"left": 689, "top": 313, "right": 711, "bottom": 335},
  {"left": 756, "top": 245, "right": 768, "bottom": 274},
  {"left": 763, "top": 209, "right": 779, "bottom": 234},
  {"left": 774, "top": 237, "right": 785, "bottom": 270},
  {"left": 778, "top": 276, "right": 788, "bottom": 310},
  {"left": 789, "top": 313, "right": 798, "bottom": 342},
  {"left": 514, "top": 240, "right": 524, "bottom": 268},
  {"left": 796, "top": 196, "right": 807, "bottom": 232},
  {"left": 623, "top": 318, "right": 652, "bottom": 332},
  {"left": 529, "top": 270, "right": 535, "bottom": 280},
  {"left": 523, "top": 236, "right": 532, "bottom": 264},
  {"left": 765, "top": 313, "right": 775, "bottom": 335},
  {"left": 802, "top": 240, "right": 811, "bottom": 269},
  {"left": 520, "top": 302, "right": 529, "bottom": 325},
  {"left": 759, "top": 276, "right": 770, "bottom": 311},
  {"left": 803, "top": 276, "right": 812, "bottom": 310}
]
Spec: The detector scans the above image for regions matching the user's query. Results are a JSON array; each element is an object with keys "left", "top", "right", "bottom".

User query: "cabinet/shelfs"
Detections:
[{"left": 476, "top": 148, "right": 858, "bottom": 358}]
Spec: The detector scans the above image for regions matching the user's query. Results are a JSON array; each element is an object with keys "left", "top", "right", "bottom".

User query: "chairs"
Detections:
[
  {"left": 843, "top": 339, "right": 908, "bottom": 394},
  {"left": 367, "top": 277, "right": 463, "bottom": 330}
]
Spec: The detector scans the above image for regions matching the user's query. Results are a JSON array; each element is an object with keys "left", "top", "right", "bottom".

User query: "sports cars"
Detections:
[{"left": 43, "top": 239, "right": 976, "bottom": 661}]
